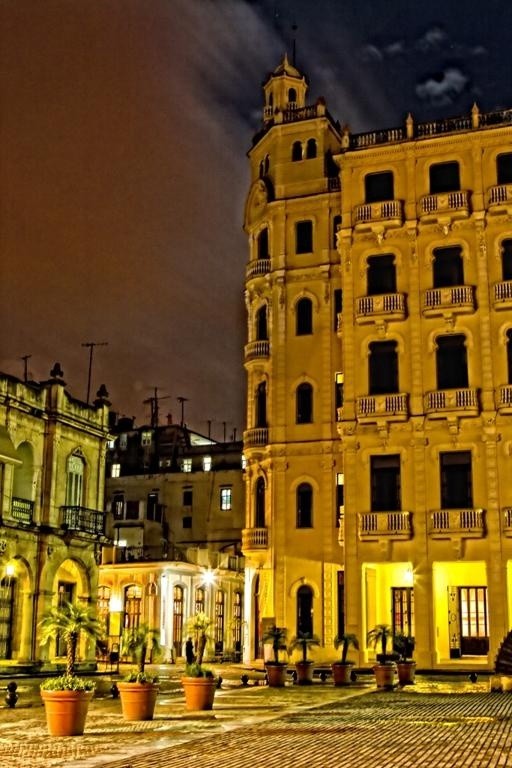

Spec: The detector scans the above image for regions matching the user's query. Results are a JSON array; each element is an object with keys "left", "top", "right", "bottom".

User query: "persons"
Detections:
[{"left": 186, "top": 635, "right": 195, "bottom": 663}]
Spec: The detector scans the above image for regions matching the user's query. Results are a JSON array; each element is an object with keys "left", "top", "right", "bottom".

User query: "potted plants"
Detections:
[
  {"left": 37, "top": 595, "right": 219, "bottom": 738},
  {"left": 259, "top": 623, "right": 416, "bottom": 689}
]
